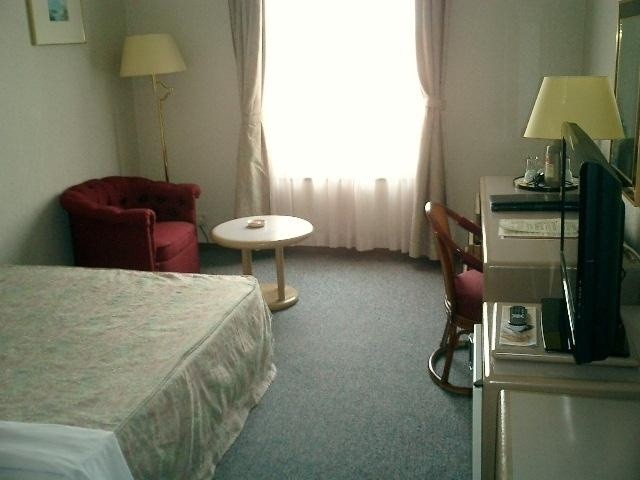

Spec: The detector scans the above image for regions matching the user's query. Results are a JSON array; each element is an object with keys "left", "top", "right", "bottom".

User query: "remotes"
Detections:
[{"left": 510, "top": 306, "right": 527, "bottom": 332}]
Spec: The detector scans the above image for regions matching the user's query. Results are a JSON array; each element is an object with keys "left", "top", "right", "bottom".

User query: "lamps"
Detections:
[
  {"left": 522, "top": 72, "right": 628, "bottom": 250},
  {"left": 119, "top": 35, "right": 188, "bottom": 181}
]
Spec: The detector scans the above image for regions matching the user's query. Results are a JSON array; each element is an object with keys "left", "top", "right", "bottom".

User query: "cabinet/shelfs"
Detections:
[{"left": 478, "top": 300, "right": 640, "bottom": 479}]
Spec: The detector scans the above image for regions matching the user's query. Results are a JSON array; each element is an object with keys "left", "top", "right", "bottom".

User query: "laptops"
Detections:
[{"left": 489, "top": 194, "right": 580, "bottom": 211}]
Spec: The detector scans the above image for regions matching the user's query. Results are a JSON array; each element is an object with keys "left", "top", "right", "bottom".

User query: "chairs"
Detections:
[
  {"left": 61, "top": 177, "right": 200, "bottom": 274},
  {"left": 425, "top": 201, "right": 485, "bottom": 394}
]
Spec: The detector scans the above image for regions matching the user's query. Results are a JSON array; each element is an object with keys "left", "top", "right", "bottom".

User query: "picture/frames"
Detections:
[
  {"left": 606, "top": 0, "right": 640, "bottom": 202},
  {"left": 25, "top": 1, "right": 86, "bottom": 45}
]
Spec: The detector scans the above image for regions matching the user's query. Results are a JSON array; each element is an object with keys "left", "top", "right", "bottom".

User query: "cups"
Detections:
[{"left": 526, "top": 156, "right": 538, "bottom": 183}]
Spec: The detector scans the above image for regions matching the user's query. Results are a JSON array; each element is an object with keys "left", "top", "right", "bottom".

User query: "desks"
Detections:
[
  {"left": 473, "top": 175, "right": 640, "bottom": 480},
  {"left": 208, "top": 212, "right": 314, "bottom": 313}
]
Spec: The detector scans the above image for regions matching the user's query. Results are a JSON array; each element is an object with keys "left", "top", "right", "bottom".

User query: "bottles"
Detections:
[{"left": 544, "top": 146, "right": 555, "bottom": 186}]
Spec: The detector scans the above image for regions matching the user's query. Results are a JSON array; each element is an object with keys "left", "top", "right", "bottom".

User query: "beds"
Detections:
[{"left": 2, "top": 265, "right": 277, "bottom": 479}]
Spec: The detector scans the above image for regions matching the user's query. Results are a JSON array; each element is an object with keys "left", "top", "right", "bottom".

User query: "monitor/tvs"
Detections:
[{"left": 540, "top": 121, "right": 630, "bottom": 365}]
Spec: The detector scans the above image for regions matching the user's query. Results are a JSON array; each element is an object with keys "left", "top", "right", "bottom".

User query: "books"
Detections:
[
  {"left": 501, "top": 303, "right": 538, "bottom": 348},
  {"left": 498, "top": 217, "right": 579, "bottom": 239}
]
